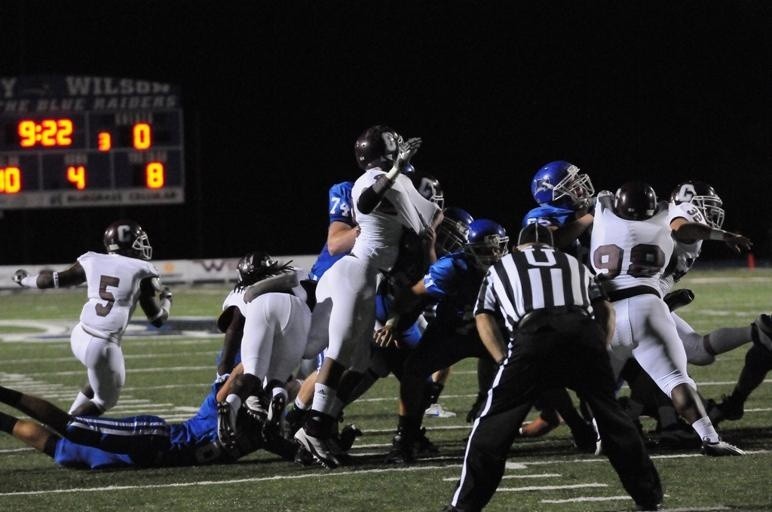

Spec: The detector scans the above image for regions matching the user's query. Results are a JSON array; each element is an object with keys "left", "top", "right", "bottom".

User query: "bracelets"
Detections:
[
  {"left": 158, "top": 298, "right": 172, "bottom": 316},
  {"left": 19, "top": 272, "right": 43, "bottom": 290}
]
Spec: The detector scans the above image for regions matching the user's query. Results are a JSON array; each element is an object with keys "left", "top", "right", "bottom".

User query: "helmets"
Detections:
[
  {"left": 418, "top": 178, "right": 474, "bottom": 256},
  {"left": 463, "top": 219, "right": 510, "bottom": 271},
  {"left": 354, "top": 124, "right": 399, "bottom": 171},
  {"left": 616, "top": 183, "right": 657, "bottom": 220},
  {"left": 524, "top": 207, "right": 570, "bottom": 243},
  {"left": 236, "top": 250, "right": 275, "bottom": 281},
  {"left": 669, "top": 177, "right": 724, "bottom": 230},
  {"left": 103, "top": 221, "right": 152, "bottom": 260},
  {"left": 531, "top": 161, "right": 595, "bottom": 210}
]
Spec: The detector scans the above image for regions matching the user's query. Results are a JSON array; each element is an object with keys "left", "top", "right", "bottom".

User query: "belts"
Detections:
[{"left": 518, "top": 305, "right": 590, "bottom": 327}]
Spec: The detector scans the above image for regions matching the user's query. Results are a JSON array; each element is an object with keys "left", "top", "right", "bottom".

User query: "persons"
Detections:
[
  {"left": 1, "top": 346, "right": 249, "bottom": 470},
  {"left": 445, "top": 219, "right": 661, "bottom": 512},
  {"left": 10, "top": 217, "right": 174, "bottom": 418},
  {"left": 215, "top": 124, "right": 772, "bottom": 473}
]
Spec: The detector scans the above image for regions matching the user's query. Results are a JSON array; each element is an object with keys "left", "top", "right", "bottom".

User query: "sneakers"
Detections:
[
  {"left": 288, "top": 406, "right": 339, "bottom": 469},
  {"left": 242, "top": 396, "right": 266, "bottom": 421},
  {"left": 426, "top": 404, "right": 456, "bottom": 419},
  {"left": 701, "top": 436, "right": 744, "bottom": 458},
  {"left": 395, "top": 428, "right": 439, "bottom": 455},
  {"left": 261, "top": 391, "right": 286, "bottom": 448},
  {"left": 215, "top": 400, "right": 240, "bottom": 457},
  {"left": 660, "top": 425, "right": 697, "bottom": 446}
]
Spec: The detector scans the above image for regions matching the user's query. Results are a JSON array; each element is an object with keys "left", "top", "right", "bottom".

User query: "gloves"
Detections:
[
  {"left": 13, "top": 268, "right": 28, "bottom": 286},
  {"left": 161, "top": 286, "right": 175, "bottom": 301},
  {"left": 726, "top": 233, "right": 753, "bottom": 254}
]
